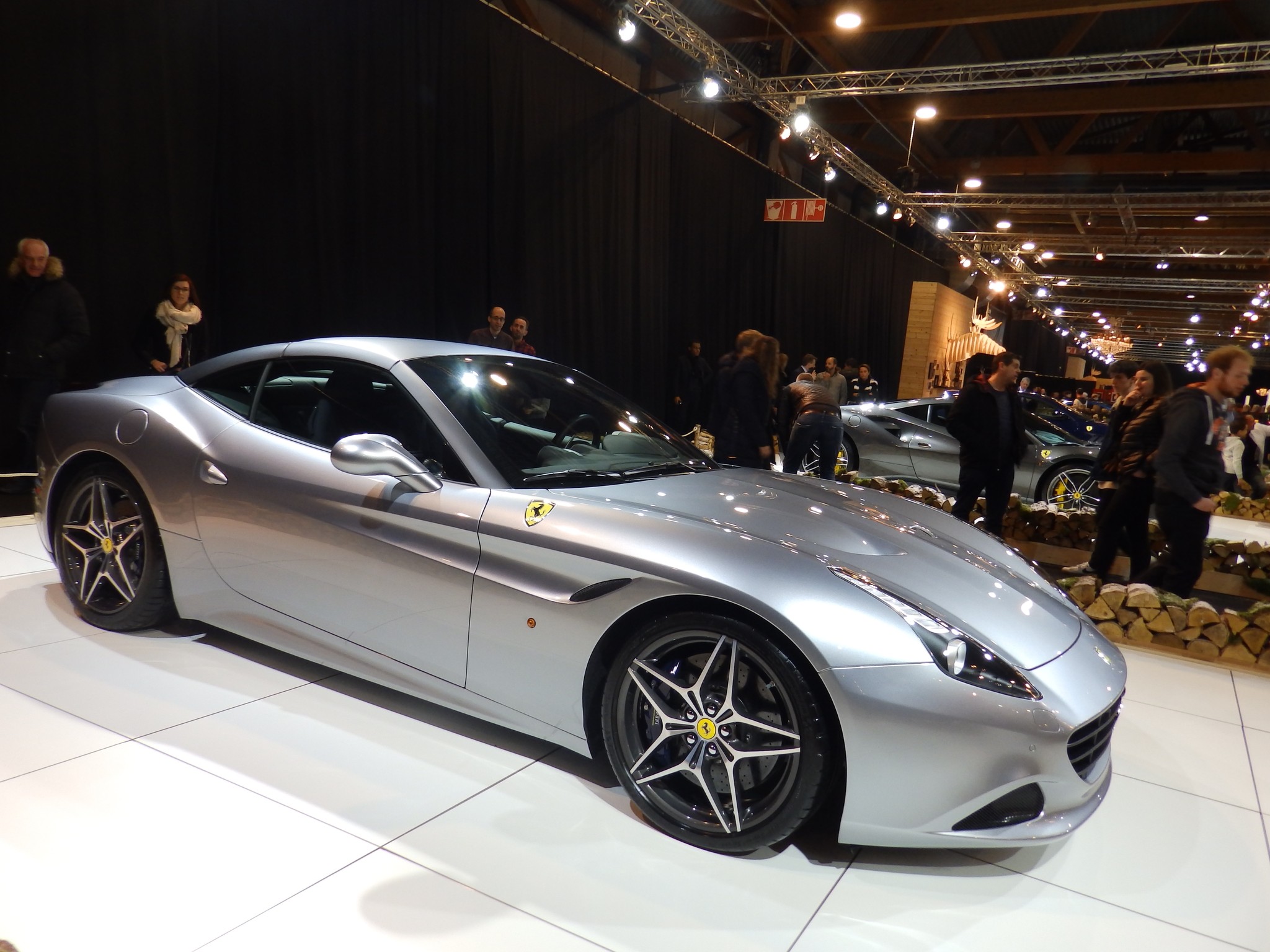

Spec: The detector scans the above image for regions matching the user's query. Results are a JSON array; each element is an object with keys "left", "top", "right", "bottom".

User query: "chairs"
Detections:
[
  {"left": 317, "top": 377, "right": 450, "bottom": 476},
  {"left": 444, "top": 387, "right": 526, "bottom": 479}
]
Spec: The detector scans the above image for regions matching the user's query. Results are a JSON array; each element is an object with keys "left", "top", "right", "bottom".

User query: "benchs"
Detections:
[{"left": 261, "top": 374, "right": 399, "bottom": 437}]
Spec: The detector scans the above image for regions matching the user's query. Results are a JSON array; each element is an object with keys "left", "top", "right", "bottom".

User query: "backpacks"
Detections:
[{"left": 1118, "top": 393, "right": 1176, "bottom": 481}]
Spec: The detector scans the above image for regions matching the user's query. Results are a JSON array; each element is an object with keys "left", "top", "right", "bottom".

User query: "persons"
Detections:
[
  {"left": 135, "top": 271, "right": 216, "bottom": 382},
  {"left": 779, "top": 312, "right": 1270, "bottom": 606},
  {"left": 671, "top": 339, "right": 711, "bottom": 452},
  {"left": 727, "top": 334, "right": 779, "bottom": 473},
  {"left": 466, "top": 305, "right": 517, "bottom": 353},
  {"left": 711, "top": 328, "right": 764, "bottom": 467},
  {"left": 505, "top": 315, "right": 536, "bottom": 358},
  {"left": 0, "top": 237, "right": 88, "bottom": 507}
]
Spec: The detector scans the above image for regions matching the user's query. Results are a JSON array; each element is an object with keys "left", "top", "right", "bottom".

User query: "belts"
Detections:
[{"left": 800, "top": 409, "right": 845, "bottom": 420}]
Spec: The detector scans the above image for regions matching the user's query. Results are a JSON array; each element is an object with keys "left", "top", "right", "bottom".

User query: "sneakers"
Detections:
[{"left": 1060, "top": 562, "right": 1099, "bottom": 577}]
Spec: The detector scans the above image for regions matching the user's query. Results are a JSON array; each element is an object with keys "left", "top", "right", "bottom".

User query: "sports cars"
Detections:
[
  {"left": 940, "top": 386, "right": 1110, "bottom": 451},
  {"left": 33, "top": 333, "right": 1126, "bottom": 851},
  {"left": 796, "top": 396, "right": 1120, "bottom": 513}
]
